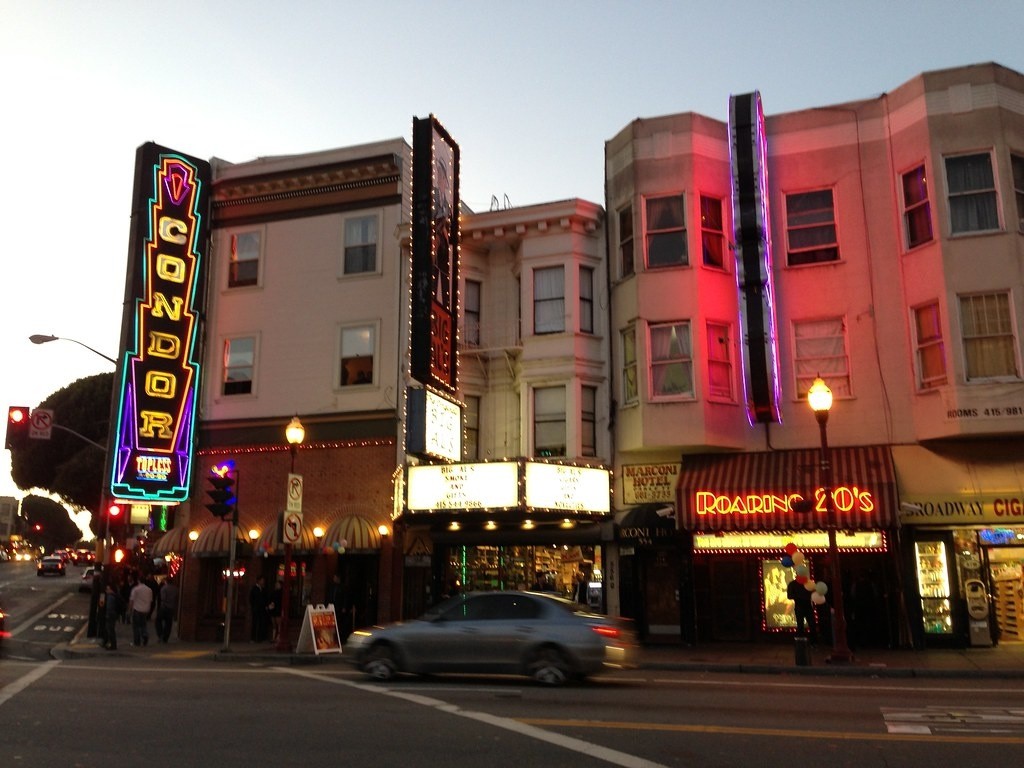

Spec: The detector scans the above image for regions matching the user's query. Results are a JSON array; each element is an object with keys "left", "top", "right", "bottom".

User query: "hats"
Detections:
[{"left": 536, "top": 571, "right": 547, "bottom": 577}]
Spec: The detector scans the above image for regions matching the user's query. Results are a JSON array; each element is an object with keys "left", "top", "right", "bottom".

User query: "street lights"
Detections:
[
  {"left": 276, "top": 412, "right": 304, "bottom": 651},
  {"left": 30, "top": 334, "right": 116, "bottom": 637},
  {"left": 808, "top": 377, "right": 851, "bottom": 662}
]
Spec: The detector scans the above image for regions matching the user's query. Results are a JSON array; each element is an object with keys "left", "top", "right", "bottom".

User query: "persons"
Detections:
[
  {"left": 99, "top": 582, "right": 119, "bottom": 651},
  {"left": 787, "top": 580, "right": 816, "bottom": 649},
  {"left": 127, "top": 575, "right": 153, "bottom": 648},
  {"left": 572, "top": 571, "right": 591, "bottom": 607},
  {"left": 530, "top": 571, "right": 555, "bottom": 591},
  {"left": 85, "top": 565, "right": 179, "bottom": 625},
  {"left": 266, "top": 580, "right": 284, "bottom": 643},
  {"left": 155, "top": 575, "right": 179, "bottom": 643},
  {"left": 330, "top": 572, "right": 347, "bottom": 644},
  {"left": 248, "top": 573, "right": 266, "bottom": 644}
]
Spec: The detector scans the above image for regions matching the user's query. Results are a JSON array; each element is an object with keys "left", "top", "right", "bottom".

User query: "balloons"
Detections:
[
  {"left": 781, "top": 543, "right": 827, "bottom": 605},
  {"left": 259, "top": 541, "right": 275, "bottom": 558},
  {"left": 320, "top": 539, "right": 348, "bottom": 554}
]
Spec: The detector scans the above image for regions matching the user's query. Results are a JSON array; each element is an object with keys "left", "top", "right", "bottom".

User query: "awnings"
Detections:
[
  {"left": 254, "top": 518, "right": 317, "bottom": 550},
  {"left": 676, "top": 445, "right": 904, "bottom": 529},
  {"left": 150, "top": 526, "right": 186, "bottom": 553},
  {"left": 322, "top": 514, "right": 382, "bottom": 549},
  {"left": 192, "top": 520, "right": 251, "bottom": 552}
]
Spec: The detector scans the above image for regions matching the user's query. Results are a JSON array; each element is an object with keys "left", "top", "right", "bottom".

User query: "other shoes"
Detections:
[
  {"left": 106, "top": 646, "right": 116, "bottom": 651},
  {"left": 98, "top": 642, "right": 107, "bottom": 648}
]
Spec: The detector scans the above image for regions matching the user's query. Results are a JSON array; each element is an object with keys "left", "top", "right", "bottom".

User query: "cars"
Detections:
[
  {"left": 38, "top": 557, "right": 64, "bottom": 576},
  {"left": 51, "top": 548, "right": 73, "bottom": 563},
  {"left": 344, "top": 591, "right": 605, "bottom": 687},
  {"left": 74, "top": 550, "right": 92, "bottom": 566},
  {"left": 521, "top": 591, "right": 639, "bottom": 670}
]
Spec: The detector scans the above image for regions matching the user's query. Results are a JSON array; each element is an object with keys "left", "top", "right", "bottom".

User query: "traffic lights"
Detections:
[
  {"left": 4, "top": 406, "right": 30, "bottom": 450},
  {"left": 206, "top": 475, "right": 234, "bottom": 518},
  {"left": 107, "top": 499, "right": 124, "bottom": 519}
]
